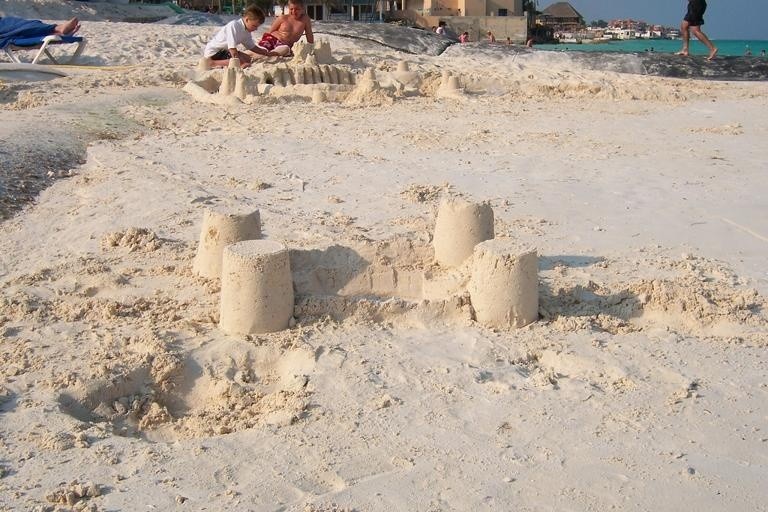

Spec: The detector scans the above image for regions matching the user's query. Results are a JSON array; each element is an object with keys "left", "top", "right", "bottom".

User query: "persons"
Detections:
[
  {"left": 506, "top": 37, "right": 512, "bottom": 44},
  {"left": 245, "top": 0, "right": 314, "bottom": 57},
  {"left": 487, "top": 31, "right": 495, "bottom": 42},
  {"left": 432, "top": 26, "right": 436, "bottom": 33},
  {"left": 203, "top": 3, "right": 271, "bottom": 69},
  {"left": 674, "top": 0, "right": 718, "bottom": 59},
  {"left": 435, "top": 22, "right": 445, "bottom": 35},
  {"left": 0, "top": 16, "right": 81, "bottom": 49},
  {"left": 527, "top": 38, "right": 533, "bottom": 47},
  {"left": 459, "top": 32, "right": 468, "bottom": 43}
]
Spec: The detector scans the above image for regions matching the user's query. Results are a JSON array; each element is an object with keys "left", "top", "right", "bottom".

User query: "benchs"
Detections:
[{"left": 0, "top": 34, "right": 91, "bottom": 68}]
